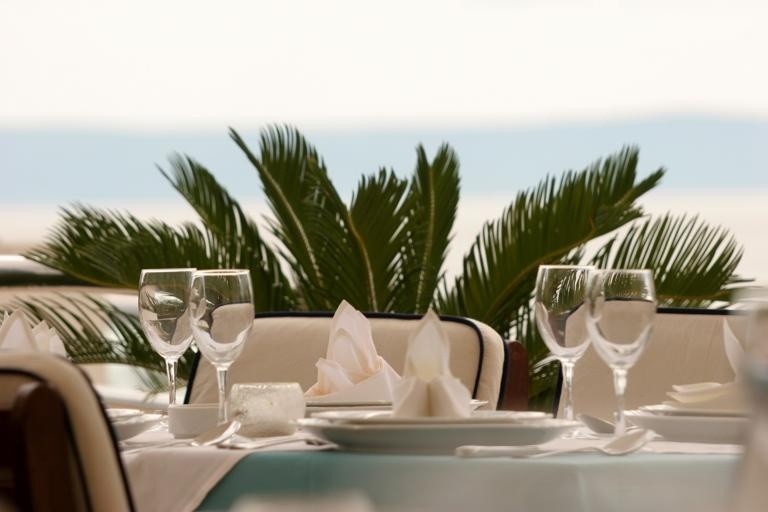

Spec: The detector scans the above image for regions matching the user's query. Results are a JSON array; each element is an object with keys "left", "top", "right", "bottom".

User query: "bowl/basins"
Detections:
[{"left": 167, "top": 402, "right": 225, "bottom": 440}]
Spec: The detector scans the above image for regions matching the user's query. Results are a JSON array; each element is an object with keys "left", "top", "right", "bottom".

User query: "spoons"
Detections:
[{"left": 457, "top": 430, "right": 653, "bottom": 455}]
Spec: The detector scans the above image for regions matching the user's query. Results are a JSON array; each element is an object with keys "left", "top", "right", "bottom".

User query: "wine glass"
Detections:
[
  {"left": 137, "top": 266, "right": 198, "bottom": 429},
  {"left": 587, "top": 269, "right": 658, "bottom": 437},
  {"left": 533, "top": 262, "right": 595, "bottom": 426},
  {"left": 188, "top": 269, "right": 256, "bottom": 427}
]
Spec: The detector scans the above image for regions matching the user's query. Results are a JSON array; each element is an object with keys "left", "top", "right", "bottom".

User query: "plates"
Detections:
[
  {"left": 613, "top": 404, "right": 757, "bottom": 444},
  {"left": 290, "top": 409, "right": 585, "bottom": 455}
]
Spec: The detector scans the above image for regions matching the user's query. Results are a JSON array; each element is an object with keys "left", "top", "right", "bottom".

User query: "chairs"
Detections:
[
  {"left": 554, "top": 301, "right": 746, "bottom": 418},
  {"left": 0, "top": 353, "right": 134, "bottom": 512},
  {"left": 181, "top": 311, "right": 506, "bottom": 413}
]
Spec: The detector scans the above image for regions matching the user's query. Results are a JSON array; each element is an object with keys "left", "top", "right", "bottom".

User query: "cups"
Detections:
[{"left": 227, "top": 380, "right": 306, "bottom": 439}]
